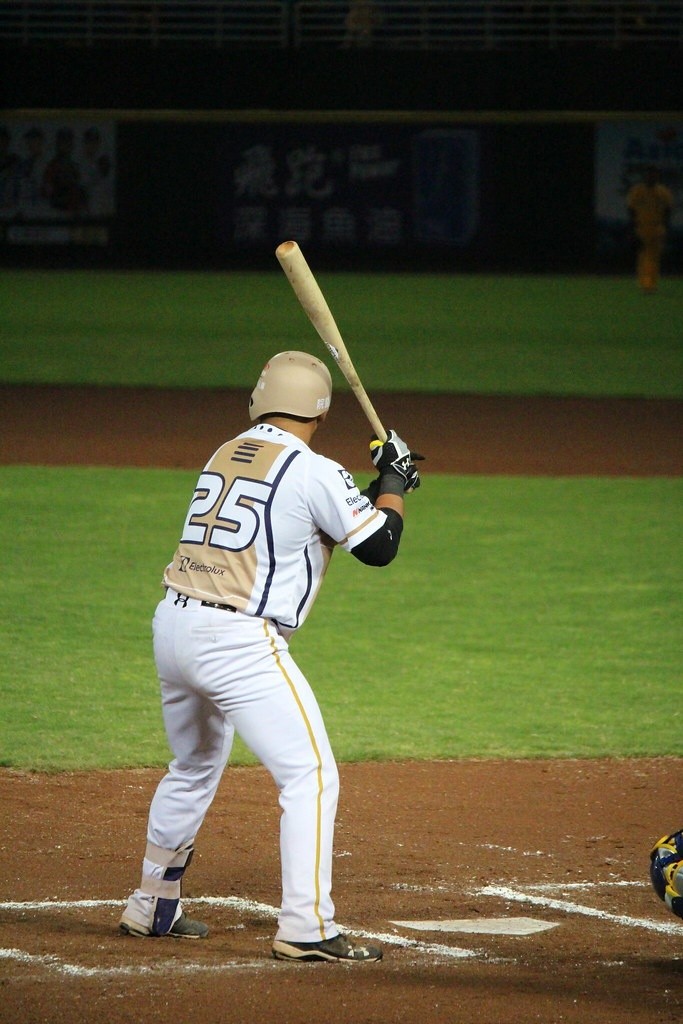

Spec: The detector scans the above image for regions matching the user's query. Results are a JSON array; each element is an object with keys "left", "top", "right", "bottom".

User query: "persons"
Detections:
[
  {"left": 626, "top": 167, "right": 674, "bottom": 294},
  {"left": 345, "top": 0, "right": 376, "bottom": 46},
  {"left": 1, "top": 125, "right": 111, "bottom": 215},
  {"left": 119, "top": 350, "right": 417, "bottom": 962}
]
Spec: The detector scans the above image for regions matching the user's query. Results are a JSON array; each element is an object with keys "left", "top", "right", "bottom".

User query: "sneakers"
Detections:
[
  {"left": 270, "top": 932, "right": 384, "bottom": 963},
  {"left": 119, "top": 909, "right": 209, "bottom": 938}
]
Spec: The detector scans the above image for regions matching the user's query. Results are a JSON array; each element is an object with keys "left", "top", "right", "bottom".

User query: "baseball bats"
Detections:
[{"left": 273, "top": 239, "right": 414, "bottom": 496}]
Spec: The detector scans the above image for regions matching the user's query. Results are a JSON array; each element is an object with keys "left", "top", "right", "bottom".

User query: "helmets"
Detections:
[{"left": 248, "top": 351, "right": 333, "bottom": 421}]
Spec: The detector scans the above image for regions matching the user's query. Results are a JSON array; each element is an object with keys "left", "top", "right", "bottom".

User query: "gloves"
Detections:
[{"left": 367, "top": 429, "right": 425, "bottom": 494}]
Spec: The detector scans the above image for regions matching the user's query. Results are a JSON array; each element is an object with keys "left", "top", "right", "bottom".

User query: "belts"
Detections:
[{"left": 198, "top": 599, "right": 236, "bottom": 613}]
